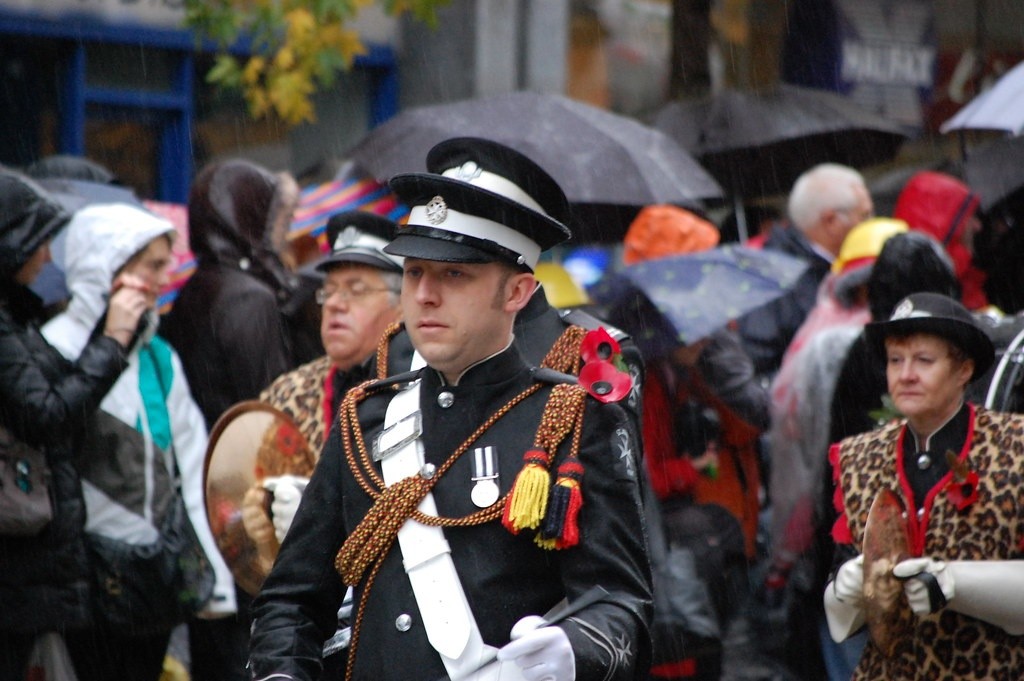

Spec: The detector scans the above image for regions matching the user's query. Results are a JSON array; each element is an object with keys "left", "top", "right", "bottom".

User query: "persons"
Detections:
[
  {"left": 644, "top": 160, "right": 1023, "bottom": 636},
  {"left": 821, "top": 293, "right": 1023, "bottom": 681},
  {"left": 0, "top": 156, "right": 773, "bottom": 681}
]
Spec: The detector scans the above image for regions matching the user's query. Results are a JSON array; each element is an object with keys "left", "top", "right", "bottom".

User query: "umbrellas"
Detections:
[
  {"left": 585, "top": 247, "right": 808, "bottom": 356},
  {"left": 355, "top": 90, "right": 724, "bottom": 245},
  {"left": 23, "top": 178, "right": 161, "bottom": 305},
  {"left": 940, "top": 62, "right": 1024, "bottom": 143},
  {"left": 632, "top": 84, "right": 910, "bottom": 245}
]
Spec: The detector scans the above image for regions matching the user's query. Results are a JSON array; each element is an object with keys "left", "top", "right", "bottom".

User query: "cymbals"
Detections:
[
  {"left": 200, "top": 398, "right": 315, "bottom": 598},
  {"left": 858, "top": 487, "right": 908, "bottom": 658}
]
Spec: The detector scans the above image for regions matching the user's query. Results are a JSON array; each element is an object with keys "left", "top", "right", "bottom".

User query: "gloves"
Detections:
[
  {"left": 834, "top": 555, "right": 866, "bottom": 605},
  {"left": 262, "top": 476, "right": 310, "bottom": 544},
  {"left": 895, "top": 557, "right": 954, "bottom": 616},
  {"left": 497, "top": 615, "right": 576, "bottom": 681}
]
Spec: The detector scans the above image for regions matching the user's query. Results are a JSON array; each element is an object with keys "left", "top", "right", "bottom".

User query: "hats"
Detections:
[
  {"left": 864, "top": 293, "right": 995, "bottom": 381},
  {"left": 314, "top": 210, "right": 405, "bottom": 274},
  {"left": 382, "top": 172, "right": 571, "bottom": 276},
  {"left": 829, "top": 216, "right": 911, "bottom": 270},
  {"left": 425, "top": 135, "right": 571, "bottom": 223}
]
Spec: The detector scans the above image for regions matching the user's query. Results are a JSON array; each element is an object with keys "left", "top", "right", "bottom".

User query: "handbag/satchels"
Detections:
[
  {"left": 83, "top": 494, "right": 216, "bottom": 636},
  {"left": 0, "top": 520, "right": 95, "bottom": 633}
]
